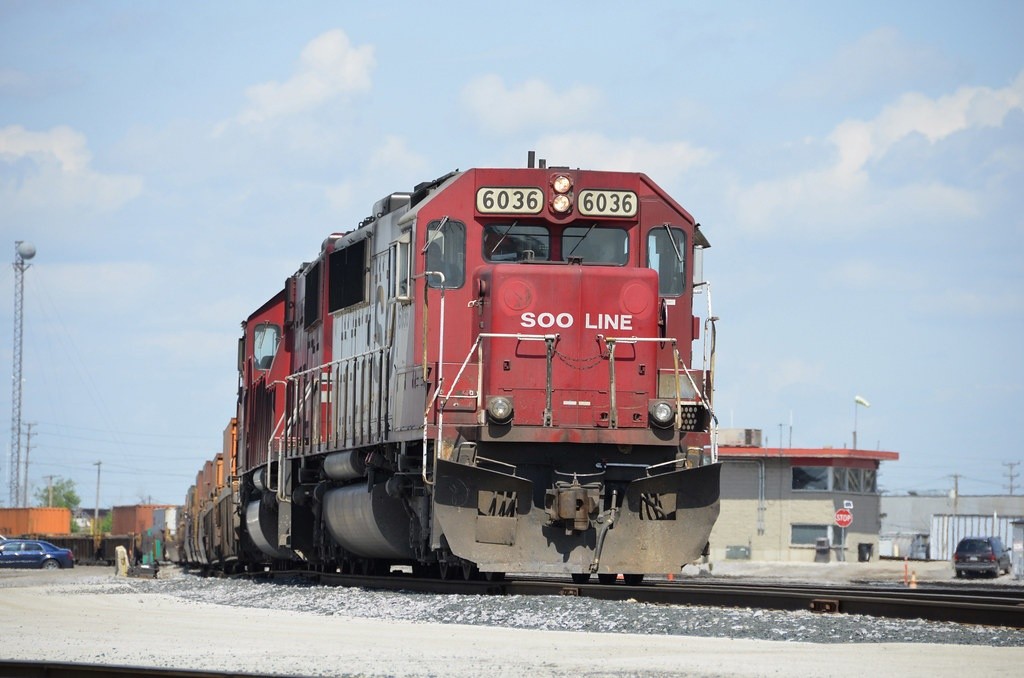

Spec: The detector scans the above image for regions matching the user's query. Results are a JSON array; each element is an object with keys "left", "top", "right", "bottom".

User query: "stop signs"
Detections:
[{"left": 835, "top": 509, "right": 853, "bottom": 527}]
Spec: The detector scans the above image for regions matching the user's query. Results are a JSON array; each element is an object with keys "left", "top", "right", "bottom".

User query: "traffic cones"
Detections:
[{"left": 909, "top": 570, "right": 918, "bottom": 589}]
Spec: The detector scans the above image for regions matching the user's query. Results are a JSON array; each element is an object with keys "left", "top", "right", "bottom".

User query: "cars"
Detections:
[
  {"left": 0, "top": 539, "right": 75, "bottom": 569},
  {"left": 0, "top": 535, "right": 7, "bottom": 542}
]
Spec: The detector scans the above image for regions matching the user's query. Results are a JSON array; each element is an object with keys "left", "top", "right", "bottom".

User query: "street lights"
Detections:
[
  {"left": 94, "top": 460, "right": 102, "bottom": 552},
  {"left": 854, "top": 395, "right": 869, "bottom": 450}
]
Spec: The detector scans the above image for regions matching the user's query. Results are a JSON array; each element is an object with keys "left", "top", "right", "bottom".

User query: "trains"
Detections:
[{"left": 171, "top": 150, "right": 724, "bottom": 583}]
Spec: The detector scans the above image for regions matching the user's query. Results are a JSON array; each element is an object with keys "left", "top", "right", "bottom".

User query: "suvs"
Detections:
[{"left": 954, "top": 530, "right": 1012, "bottom": 578}]
